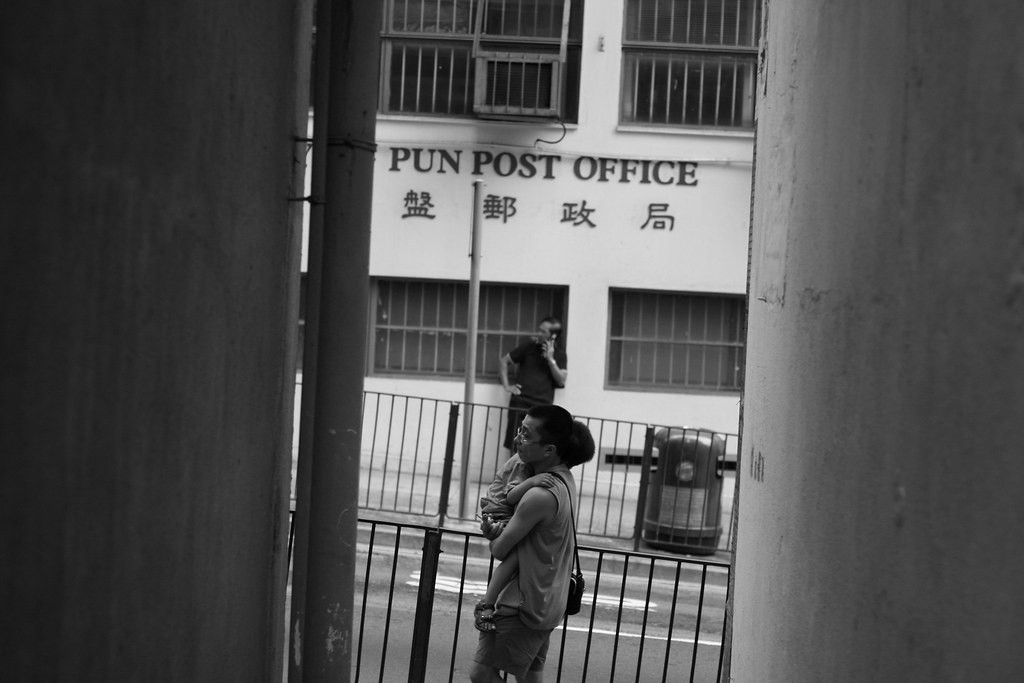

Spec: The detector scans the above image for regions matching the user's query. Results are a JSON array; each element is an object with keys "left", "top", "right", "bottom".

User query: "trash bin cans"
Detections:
[{"left": 642, "top": 427, "right": 726, "bottom": 558}]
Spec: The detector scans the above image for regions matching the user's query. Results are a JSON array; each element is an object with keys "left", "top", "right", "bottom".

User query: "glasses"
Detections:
[{"left": 517, "top": 427, "right": 547, "bottom": 446}]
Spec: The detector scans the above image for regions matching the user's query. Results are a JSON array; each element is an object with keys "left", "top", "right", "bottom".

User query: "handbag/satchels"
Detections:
[{"left": 566, "top": 573, "right": 584, "bottom": 615}]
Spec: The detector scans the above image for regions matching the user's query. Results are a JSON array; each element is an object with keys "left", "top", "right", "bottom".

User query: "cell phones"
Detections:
[{"left": 548, "top": 333, "right": 556, "bottom": 342}]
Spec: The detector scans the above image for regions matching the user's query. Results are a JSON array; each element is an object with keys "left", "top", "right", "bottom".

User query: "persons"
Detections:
[
  {"left": 498, "top": 316, "right": 568, "bottom": 459},
  {"left": 474, "top": 420, "right": 596, "bottom": 632},
  {"left": 469, "top": 404, "right": 577, "bottom": 683}
]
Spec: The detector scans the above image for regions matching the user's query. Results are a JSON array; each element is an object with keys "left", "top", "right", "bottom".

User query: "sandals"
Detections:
[{"left": 474, "top": 599, "right": 497, "bottom": 632}]
